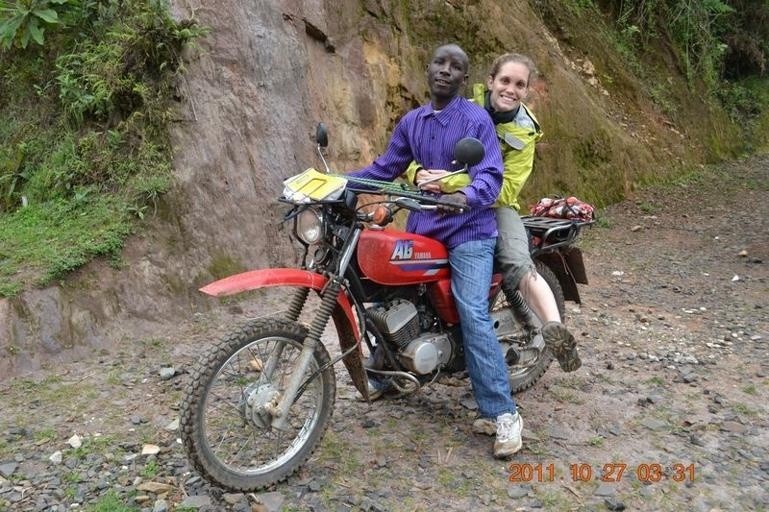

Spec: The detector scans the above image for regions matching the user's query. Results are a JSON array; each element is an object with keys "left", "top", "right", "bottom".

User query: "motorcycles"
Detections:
[{"left": 176, "top": 118, "right": 598, "bottom": 496}]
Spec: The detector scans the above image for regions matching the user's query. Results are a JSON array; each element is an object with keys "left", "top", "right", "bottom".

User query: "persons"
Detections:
[
  {"left": 404, "top": 51, "right": 583, "bottom": 374},
  {"left": 334, "top": 41, "right": 526, "bottom": 460}
]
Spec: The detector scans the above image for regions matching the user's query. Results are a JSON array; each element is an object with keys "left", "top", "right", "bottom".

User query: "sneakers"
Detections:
[
  {"left": 493, "top": 410, "right": 524, "bottom": 458},
  {"left": 355, "top": 380, "right": 382, "bottom": 401},
  {"left": 542, "top": 323, "right": 581, "bottom": 372}
]
